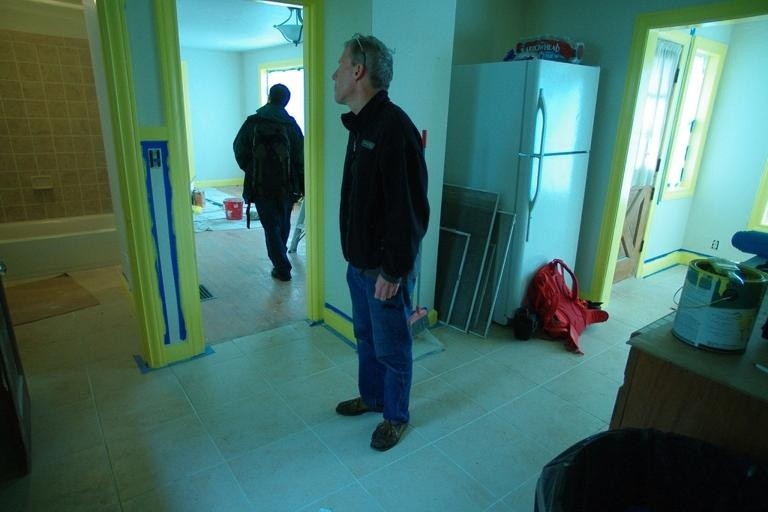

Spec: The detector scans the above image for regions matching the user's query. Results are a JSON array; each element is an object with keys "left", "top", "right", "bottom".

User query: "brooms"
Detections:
[{"left": 406, "top": 129, "right": 430, "bottom": 334}]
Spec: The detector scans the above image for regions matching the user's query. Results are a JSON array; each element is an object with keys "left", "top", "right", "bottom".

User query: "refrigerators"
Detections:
[{"left": 439, "top": 58, "right": 602, "bottom": 329}]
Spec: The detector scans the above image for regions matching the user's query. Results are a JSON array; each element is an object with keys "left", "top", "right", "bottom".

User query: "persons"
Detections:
[
  {"left": 331, "top": 36, "right": 430, "bottom": 452},
  {"left": 233, "top": 83, "right": 304, "bottom": 281}
]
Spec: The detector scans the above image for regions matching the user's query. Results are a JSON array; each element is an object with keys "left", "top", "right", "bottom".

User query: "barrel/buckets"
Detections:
[
  {"left": 223, "top": 197, "right": 244, "bottom": 219},
  {"left": 671, "top": 258, "right": 768, "bottom": 355},
  {"left": 194, "top": 190, "right": 206, "bottom": 207}
]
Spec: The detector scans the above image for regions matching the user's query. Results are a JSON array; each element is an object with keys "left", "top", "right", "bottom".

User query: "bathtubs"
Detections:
[{"left": 0, "top": 212, "right": 123, "bottom": 282}]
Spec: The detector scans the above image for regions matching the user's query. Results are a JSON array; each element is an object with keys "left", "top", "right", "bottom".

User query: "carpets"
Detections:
[{"left": 4, "top": 272, "right": 102, "bottom": 327}]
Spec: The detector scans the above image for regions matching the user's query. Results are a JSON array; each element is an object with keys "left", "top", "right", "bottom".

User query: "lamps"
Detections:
[{"left": 272, "top": 5, "right": 305, "bottom": 48}]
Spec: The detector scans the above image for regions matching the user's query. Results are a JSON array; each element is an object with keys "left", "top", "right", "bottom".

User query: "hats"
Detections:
[{"left": 270, "top": 83, "right": 291, "bottom": 104}]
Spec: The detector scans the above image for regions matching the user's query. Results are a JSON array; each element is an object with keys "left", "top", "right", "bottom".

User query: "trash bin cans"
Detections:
[{"left": 536, "top": 429, "right": 767, "bottom": 511}]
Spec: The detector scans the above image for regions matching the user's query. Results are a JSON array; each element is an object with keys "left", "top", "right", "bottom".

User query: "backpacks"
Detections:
[
  {"left": 248, "top": 117, "right": 291, "bottom": 201},
  {"left": 531, "top": 259, "right": 610, "bottom": 355}
]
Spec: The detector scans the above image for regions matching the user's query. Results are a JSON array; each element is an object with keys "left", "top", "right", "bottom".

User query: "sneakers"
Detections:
[
  {"left": 336, "top": 396, "right": 385, "bottom": 416},
  {"left": 371, "top": 418, "right": 407, "bottom": 451},
  {"left": 271, "top": 268, "right": 292, "bottom": 281}
]
